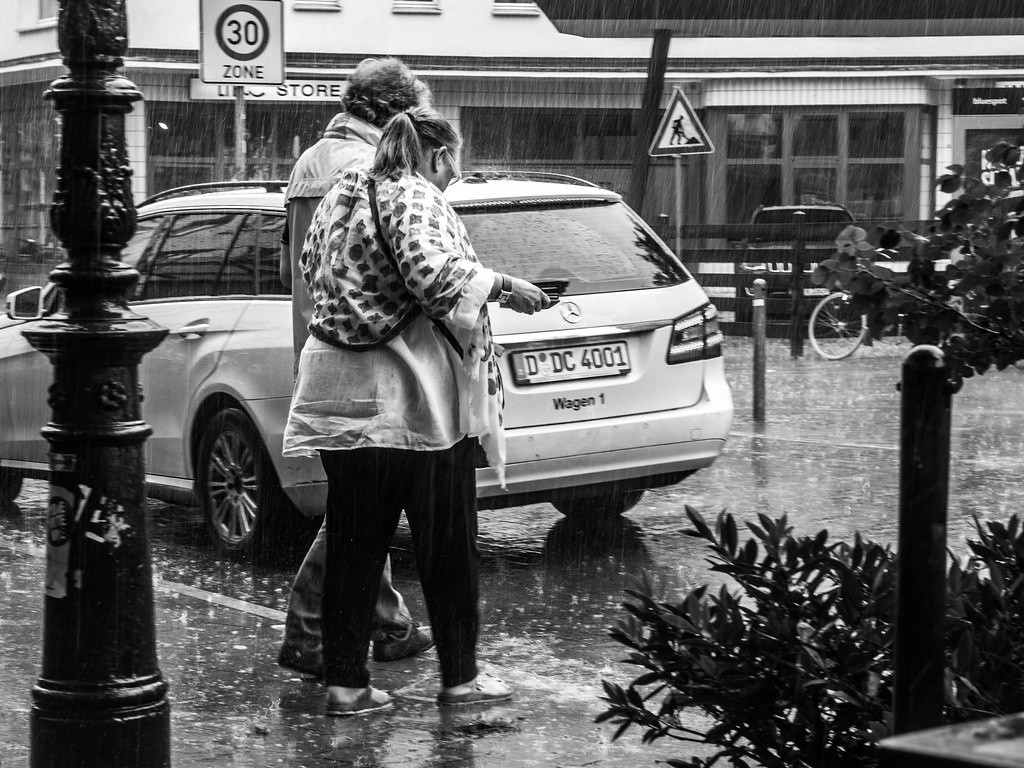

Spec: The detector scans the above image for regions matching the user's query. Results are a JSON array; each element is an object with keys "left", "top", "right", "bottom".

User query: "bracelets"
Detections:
[{"left": 496, "top": 274, "right": 512, "bottom": 304}]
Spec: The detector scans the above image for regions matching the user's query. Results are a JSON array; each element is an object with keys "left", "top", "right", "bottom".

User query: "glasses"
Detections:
[{"left": 433, "top": 148, "right": 462, "bottom": 186}]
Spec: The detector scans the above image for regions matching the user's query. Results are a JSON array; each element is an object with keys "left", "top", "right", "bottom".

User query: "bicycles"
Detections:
[{"left": 808, "top": 247, "right": 900, "bottom": 360}]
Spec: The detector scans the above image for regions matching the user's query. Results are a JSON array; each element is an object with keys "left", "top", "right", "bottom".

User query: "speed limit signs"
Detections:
[{"left": 199, "top": 0, "right": 288, "bottom": 86}]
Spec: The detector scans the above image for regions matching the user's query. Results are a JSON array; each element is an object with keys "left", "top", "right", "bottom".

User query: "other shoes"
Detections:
[
  {"left": 438, "top": 679, "right": 513, "bottom": 706},
  {"left": 326, "top": 686, "right": 393, "bottom": 715}
]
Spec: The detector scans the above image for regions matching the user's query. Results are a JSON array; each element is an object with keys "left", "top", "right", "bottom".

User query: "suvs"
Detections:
[
  {"left": 737, "top": 204, "right": 863, "bottom": 321},
  {"left": 0, "top": 171, "right": 737, "bottom": 563}
]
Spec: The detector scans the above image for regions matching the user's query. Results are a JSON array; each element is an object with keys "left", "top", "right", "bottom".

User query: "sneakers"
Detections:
[
  {"left": 277, "top": 635, "right": 325, "bottom": 676},
  {"left": 373, "top": 625, "right": 435, "bottom": 662}
]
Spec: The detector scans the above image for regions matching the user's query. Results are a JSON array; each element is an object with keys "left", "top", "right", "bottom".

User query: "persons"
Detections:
[
  {"left": 276, "top": 58, "right": 433, "bottom": 677},
  {"left": 282, "top": 108, "right": 551, "bottom": 716}
]
